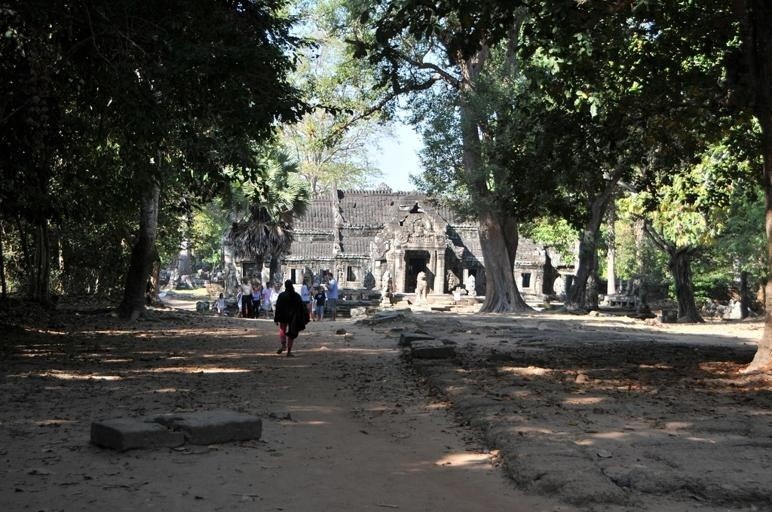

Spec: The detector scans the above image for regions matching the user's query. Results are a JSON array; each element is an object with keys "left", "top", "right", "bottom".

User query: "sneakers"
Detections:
[
  {"left": 287, "top": 353, "right": 294, "bottom": 357},
  {"left": 276, "top": 347, "right": 286, "bottom": 354}
]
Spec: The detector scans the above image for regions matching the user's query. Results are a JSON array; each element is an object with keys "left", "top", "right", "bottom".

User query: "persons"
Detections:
[
  {"left": 274, "top": 279, "right": 303, "bottom": 358},
  {"left": 216, "top": 292, "right": 225, "bottom": 317},
  {"left": 324, "top": 272, "right": 339, "bottom": 322},
  {"left": 236, "top": 276, "right": 326, "bottom": 323}
]
